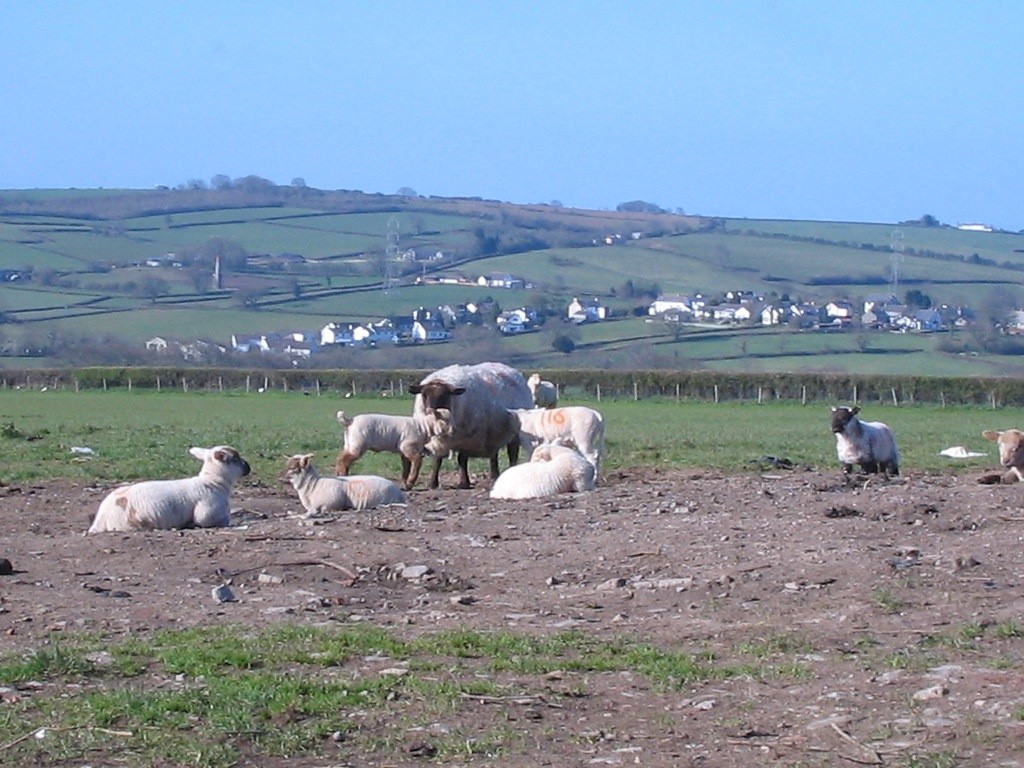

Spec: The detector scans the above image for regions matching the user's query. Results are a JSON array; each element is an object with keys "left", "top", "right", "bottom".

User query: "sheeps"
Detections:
[
  {"left": 84, "top": 442, "right": 250, "bottom": 536},
  {"left": 284, "top": 360, "right": 605, "bottom": 519},
  {"left": 829, "top": 401, "right": 900, "bottom": 476},
  {"left": 979, "top": 422, "right": 1024, "bottom": 485}
]
